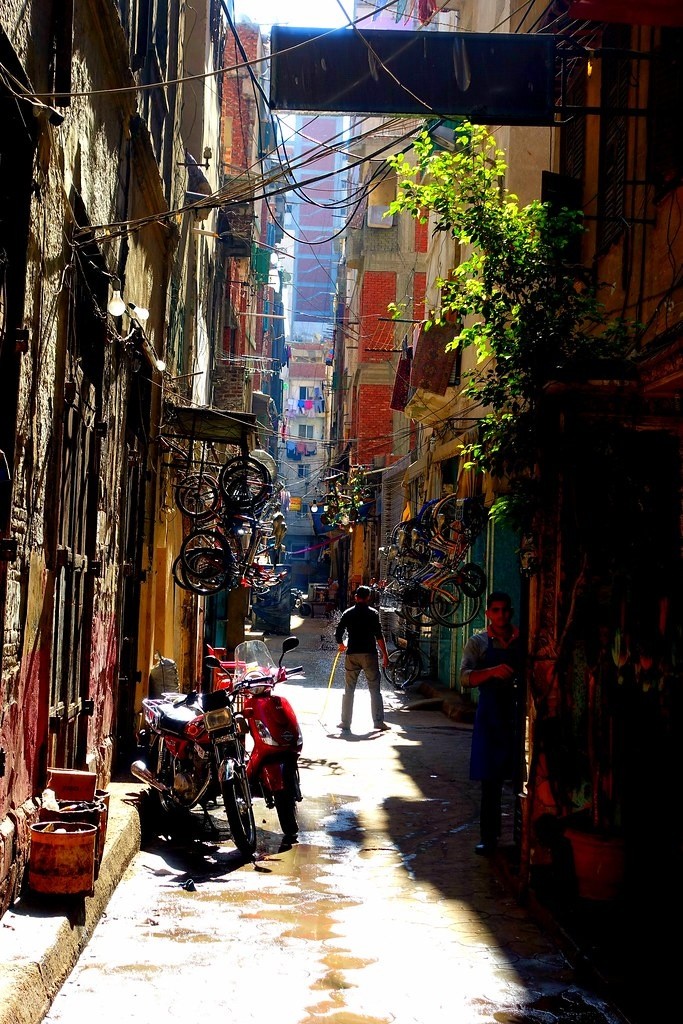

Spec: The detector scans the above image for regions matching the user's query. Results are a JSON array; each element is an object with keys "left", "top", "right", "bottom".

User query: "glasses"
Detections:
[{"left": 490, "top": 607, "right": 510, "bottom": 612}]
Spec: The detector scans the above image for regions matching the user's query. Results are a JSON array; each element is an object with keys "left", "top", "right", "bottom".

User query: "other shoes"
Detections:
[
  {"left": 373, "top": 722, "right": 390, "bottom": 731},
  {"left": 477, "top": 834, "right": 497, "bottom": 852},
  {"left": 338, "top": 722, "right": 351, "bottom": 728}
]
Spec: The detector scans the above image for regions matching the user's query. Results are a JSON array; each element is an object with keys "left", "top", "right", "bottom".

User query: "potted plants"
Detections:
[{"left": 564, "top": 625, "right": 624, "bottom": 899}]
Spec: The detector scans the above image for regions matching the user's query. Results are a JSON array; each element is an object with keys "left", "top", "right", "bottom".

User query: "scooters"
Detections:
[
  {"left": 291, "top": 589, "right": 311, "bottom": 616},
  {"left": 204, "top": 635, "right": 305, "bottom": 835}
]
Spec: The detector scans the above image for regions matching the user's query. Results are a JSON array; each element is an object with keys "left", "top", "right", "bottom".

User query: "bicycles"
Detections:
[
  {"left": 384, "top": 624, "right": 453, "bottom": 689},
  {"left": 171, "top": 456, "right": 281, "bottom": 595},
  {"left": 376, "top": 491, "right": 486, "bottom": 628}
]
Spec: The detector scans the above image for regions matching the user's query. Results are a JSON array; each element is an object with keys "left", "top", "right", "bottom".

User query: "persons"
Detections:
[
  {"left": 459, "top": 592, "right": 525, "bottom": 855},
  {"left": 336, "top": 586, "right": 391, "bottom": 730}
]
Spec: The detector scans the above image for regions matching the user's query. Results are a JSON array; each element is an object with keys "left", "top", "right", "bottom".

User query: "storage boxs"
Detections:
[{"left": 47, "top": 767, "right": 97, "bottom": 802}]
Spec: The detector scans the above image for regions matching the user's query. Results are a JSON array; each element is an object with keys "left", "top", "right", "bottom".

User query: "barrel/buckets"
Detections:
[{"left": 28, "top": 789, "right": 111, "bottom": 902}]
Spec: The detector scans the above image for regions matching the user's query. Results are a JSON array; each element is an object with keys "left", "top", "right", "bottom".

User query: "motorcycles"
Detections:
[{"left": 129, "top": 677, "right": 271, "bottom": 854}]
